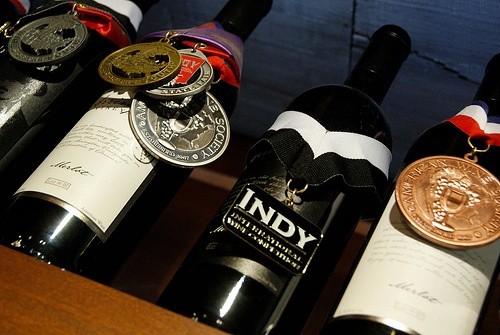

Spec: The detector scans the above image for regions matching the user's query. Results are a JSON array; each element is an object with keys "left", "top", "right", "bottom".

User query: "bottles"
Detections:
[
  {"left": 1, "top": 0, "right": 159, "bottom": 233},
  {"left": 318, "top": 52, "right": 500, "bottom": 335},
  {"left": 1, "top": 0, "right": 274, "bottom": 283},
  {"left": 153, "top": 24, "right": 412, "bottom": 335}
]
